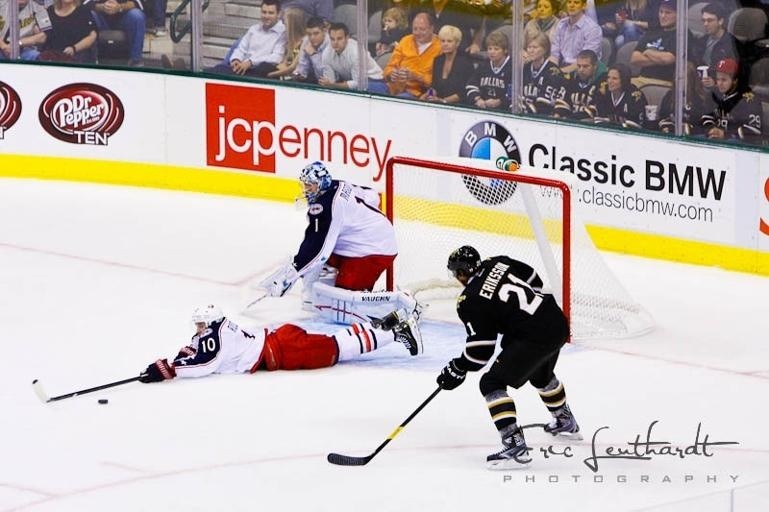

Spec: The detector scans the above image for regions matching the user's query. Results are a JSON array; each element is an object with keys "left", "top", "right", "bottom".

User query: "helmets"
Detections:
[
  {"left": 192, "top": 304, "right": 224, "bottom": 328},
  {"left": 299, "top": 161, "right": 334, "bottom": 204},
  {"left": 447, "top": 245, "right": 482, "bottom": 277},
  {"left": 715, "top": 58, "right": 739, "bottom": 74}
]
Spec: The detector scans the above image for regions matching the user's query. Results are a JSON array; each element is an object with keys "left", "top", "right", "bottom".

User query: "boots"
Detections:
[
  {"left": 486, "top": 426, "right": 529, "bottom": 461},
  {"left": 370, "top": 308, "right": 419, "bottom": 355},
  {"left": 545, "top": 406, "right": 580, "bottom": 435}
]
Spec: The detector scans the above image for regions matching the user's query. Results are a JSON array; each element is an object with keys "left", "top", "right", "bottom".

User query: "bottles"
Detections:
[{"left": 496, "top": 157, "right": 520, "bottom": 172}]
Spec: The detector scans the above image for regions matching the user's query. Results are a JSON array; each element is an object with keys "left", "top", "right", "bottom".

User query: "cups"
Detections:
[
  {"left": 643, "top": 104, "right": 658, "bottom": 122},
  {"left": 696, "top": 66, "right": 708, "bottom": 82}
]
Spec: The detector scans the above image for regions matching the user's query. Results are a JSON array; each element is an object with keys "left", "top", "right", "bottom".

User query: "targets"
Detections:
[
  {"left": 33, "top": 377, "right": 140, "bottom": 403},
  {"left": 328, "top": 387, "right": 442, "bottom": 466}
]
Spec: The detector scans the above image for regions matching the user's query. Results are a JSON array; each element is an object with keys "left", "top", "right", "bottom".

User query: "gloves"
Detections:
[
  {"left": 173, "top": 346, "right": 196, "bottom": 361},
  {"left": 436, "top": 357, "right": 467, "bottom": 390},
  {"left": 138, "top": 359, "right": 177, "bottom": 384}
]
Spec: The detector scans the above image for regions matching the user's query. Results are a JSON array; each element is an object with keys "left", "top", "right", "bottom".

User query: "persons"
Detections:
[
  {"left": 436, "top": 246, "right": 579, "bottom": 462},
  {"left": 266, "top": 162, "right": 404, "bottom": 324},
  {"left": 142, "top": 305, "right": 418, "bottom": 382},
  {"left": 1, "top": 1, "right": 768, "bottom": 144}
]
